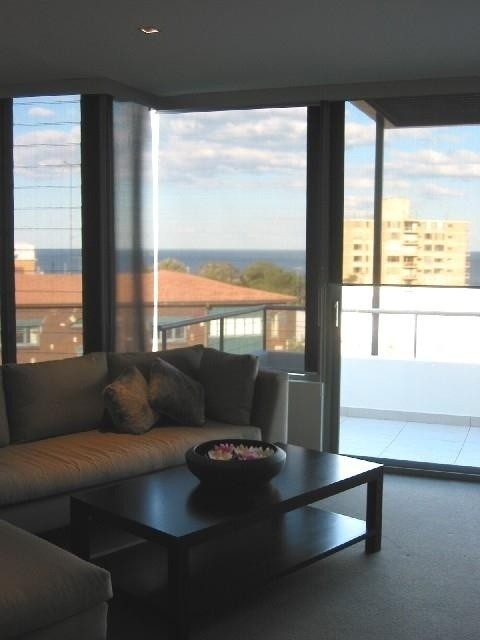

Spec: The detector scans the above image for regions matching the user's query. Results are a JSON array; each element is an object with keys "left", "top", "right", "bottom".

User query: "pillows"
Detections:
[
  {"left": 101, "top": 363, "right": 161, "bottom": 433},
  {"left": 200, "top": 346, "right": 258, "bottom": 427},
  {"left": 147, "top": 354, "right": 209, "bottom": 428}
]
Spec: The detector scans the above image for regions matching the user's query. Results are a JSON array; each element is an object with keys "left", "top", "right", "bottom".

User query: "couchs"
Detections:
[{"left": 0, "top": 339, "right": 292, "bottom": 640}]
